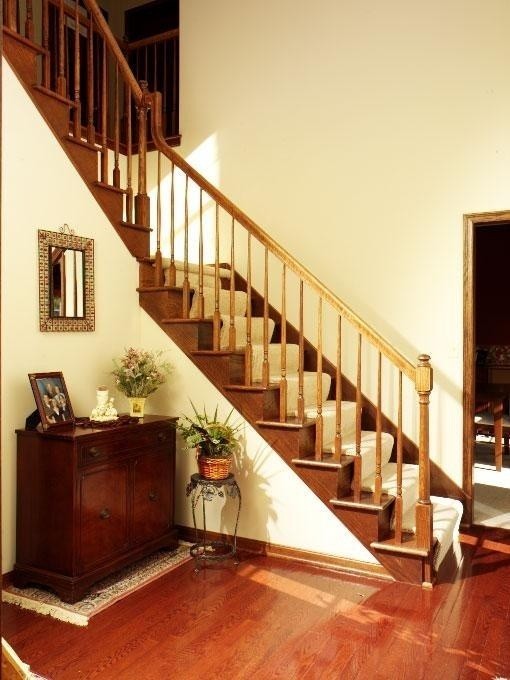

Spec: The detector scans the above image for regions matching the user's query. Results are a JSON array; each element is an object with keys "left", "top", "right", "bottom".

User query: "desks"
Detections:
[{"left": 185, "top": 473, "right": 241, "bottom": 577}]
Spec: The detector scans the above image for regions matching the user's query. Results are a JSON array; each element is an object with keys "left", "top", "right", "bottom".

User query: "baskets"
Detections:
[{"left": 191, "top": 422, "right": 234, "bottom": 481}]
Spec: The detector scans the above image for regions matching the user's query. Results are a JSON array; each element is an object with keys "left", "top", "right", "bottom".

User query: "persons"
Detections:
[{"left": 42, "top": 383, "right": 69, "bottom": 423}]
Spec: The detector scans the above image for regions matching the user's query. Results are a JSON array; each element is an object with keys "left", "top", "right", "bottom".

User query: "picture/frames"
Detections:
[{"left": 27, "top": 372, "right": 75, "bottom": 429}]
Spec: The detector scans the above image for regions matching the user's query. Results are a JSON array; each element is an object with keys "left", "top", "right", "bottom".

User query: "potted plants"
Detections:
[{"left": 166, "top": 400, "right": 248, "bottom": 481}]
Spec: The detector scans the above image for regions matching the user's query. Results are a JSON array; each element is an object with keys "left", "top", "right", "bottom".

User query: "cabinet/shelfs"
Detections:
[{"left": 14, "top": 413, "right": 180, "bottom": 605}]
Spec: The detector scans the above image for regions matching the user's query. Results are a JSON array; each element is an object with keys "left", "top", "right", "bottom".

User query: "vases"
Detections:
[{"left": 127, "top": 398, "right": 146, "bottom": 418}]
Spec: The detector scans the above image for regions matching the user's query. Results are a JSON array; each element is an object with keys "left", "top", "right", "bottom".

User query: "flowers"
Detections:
[{"left": 109, "top": 343, "right": 173, "bottom": 398}]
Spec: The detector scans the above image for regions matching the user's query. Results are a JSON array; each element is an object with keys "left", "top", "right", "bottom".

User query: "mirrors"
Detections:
[{"left": 38, "top": 224, "right": 96, "bottom": 333}]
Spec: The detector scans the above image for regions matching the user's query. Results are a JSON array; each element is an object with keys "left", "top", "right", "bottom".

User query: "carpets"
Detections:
[{"left": 1, "top": 540, "right": 198, "bottom": 627}]
